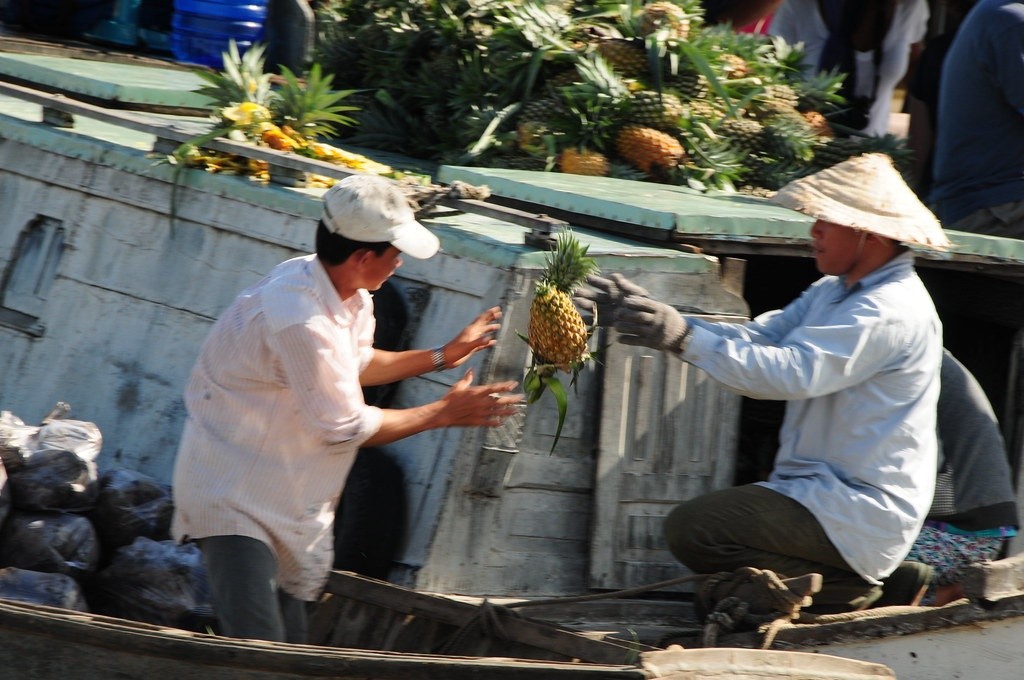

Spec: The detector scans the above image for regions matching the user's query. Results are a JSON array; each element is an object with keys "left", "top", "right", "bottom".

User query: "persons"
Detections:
[
  {"left": 905, "top": 347, "right": 1021, "bottom": 606},
  {"left": 700, "top": 0, "right": 1024, "bottom": 240},
  {"left": 170, "top": 175, "right": 524, "bottom": 644},
  {"left": 574, "top": 153, "right": 950, "bottom": 611}
]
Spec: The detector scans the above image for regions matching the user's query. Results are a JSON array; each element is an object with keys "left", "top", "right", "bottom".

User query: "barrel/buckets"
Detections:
[{"left": 167, "top": 0, "right": 272, "bottom": 71}]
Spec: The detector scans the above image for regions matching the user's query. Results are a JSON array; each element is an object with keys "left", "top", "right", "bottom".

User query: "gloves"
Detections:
[
  {"left": 613, "top": 294, "right": 694, "bottom": 355},
  {"left": 572, "top": 273, "right": 651, "bottom": 328}
]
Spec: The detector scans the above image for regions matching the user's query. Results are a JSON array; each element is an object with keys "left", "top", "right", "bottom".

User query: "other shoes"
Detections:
[{"left": 881, "top": 560, "right": 932, "bottom": 607}]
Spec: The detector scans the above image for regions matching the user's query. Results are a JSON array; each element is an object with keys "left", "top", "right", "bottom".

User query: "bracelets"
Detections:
[{"left": 431, "top": 346, "right": 445, "bottom": 373}]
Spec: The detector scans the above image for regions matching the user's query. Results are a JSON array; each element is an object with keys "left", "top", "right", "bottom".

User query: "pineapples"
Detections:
[
  {"left": 193, "top": 0, "right": 916, "bottom": 195},
  {"left": 531, "top": 226, "right": 600, "bottom": 368}
]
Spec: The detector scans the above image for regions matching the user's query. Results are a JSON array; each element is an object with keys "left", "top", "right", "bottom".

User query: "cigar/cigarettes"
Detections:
[{"left": 489, "top": 393, "right": 499, "bottom": 399}]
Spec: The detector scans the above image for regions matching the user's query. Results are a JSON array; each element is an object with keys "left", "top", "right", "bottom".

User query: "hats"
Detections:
[
  {"left": 769, "top": 153, "right": 952, "bottom": 253},
  {"left": 321, "top": 174, "right": 440, "bottom": 259}
]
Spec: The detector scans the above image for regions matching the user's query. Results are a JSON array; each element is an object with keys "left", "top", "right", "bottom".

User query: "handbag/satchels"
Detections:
[{"left": 926, "top": 462, "right": 956, "bottom": 519}]
